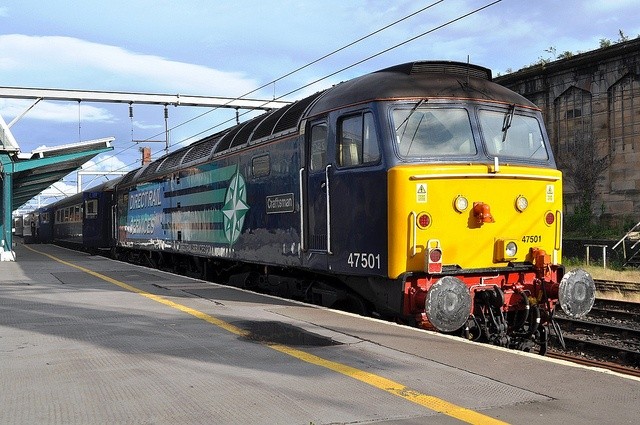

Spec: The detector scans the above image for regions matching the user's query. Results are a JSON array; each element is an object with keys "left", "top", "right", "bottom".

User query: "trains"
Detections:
[{"left": 12, "top": 60, "right": 596, "bottom": 356}]
